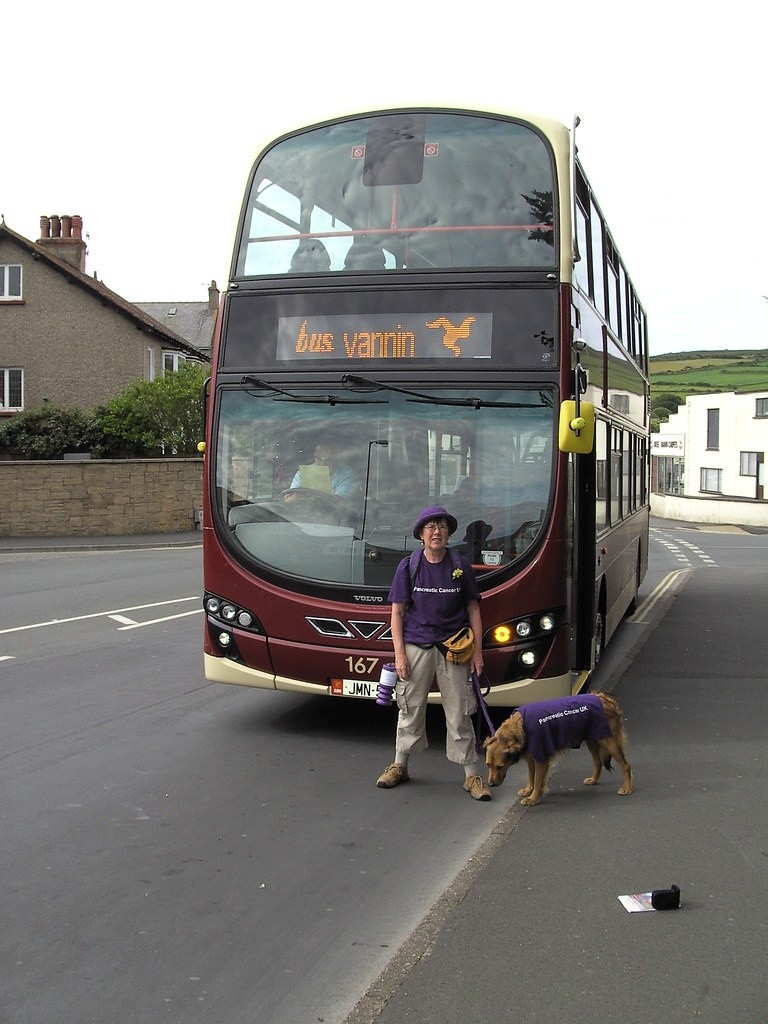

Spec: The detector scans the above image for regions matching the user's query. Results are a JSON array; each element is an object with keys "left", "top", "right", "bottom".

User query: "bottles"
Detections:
[{"left": 376, "top": 664, "right": 398, "bottom": 706}]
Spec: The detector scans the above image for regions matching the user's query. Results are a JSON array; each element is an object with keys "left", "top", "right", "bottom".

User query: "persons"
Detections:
[
  {"left": 276, "top": 238, "right": 534, "bottom": 503},
  {"left": 375, "top": 508, "right": 494, "bottom": 800}
]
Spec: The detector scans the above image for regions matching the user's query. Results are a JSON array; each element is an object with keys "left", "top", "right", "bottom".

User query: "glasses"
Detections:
[{"left": 423, "top": 524, "right": 450, "bottom": 532}]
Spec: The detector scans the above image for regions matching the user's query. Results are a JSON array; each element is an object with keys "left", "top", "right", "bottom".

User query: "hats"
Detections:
[{"left": 413, "top": 507, "right": 458, "bottom": 540}]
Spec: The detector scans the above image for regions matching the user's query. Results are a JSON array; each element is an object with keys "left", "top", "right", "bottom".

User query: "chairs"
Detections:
[
  {"left": 289, "top": 240, "right": 332, "bottom": 274},
  {"left": 342, "top": 236, "right": 388, "bottom": 270}
]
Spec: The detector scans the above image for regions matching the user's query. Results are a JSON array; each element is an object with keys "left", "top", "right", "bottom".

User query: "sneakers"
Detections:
[
  {"left": 376, "top": 762, "right": 409, "bottom": 787},
  {"left": 463, "top": 775, "right": 493, "bottom": 801}
]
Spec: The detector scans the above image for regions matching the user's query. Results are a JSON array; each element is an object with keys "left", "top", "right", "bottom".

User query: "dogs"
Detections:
[{"left": 482, "top": 690, "right": 633, "bottom": 805}]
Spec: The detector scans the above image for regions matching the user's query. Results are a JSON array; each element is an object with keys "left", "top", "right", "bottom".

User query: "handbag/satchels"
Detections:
[{"left": 441, "top": 627, "right": 476, "bottom": 665}]
